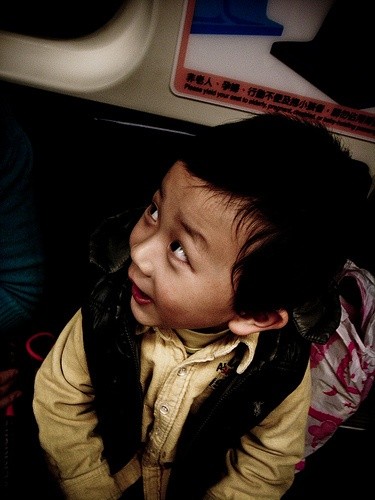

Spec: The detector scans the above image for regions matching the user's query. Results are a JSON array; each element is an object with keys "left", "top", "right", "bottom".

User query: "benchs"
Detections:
[{"left": 1, "top": 76, "right": 375, "bottom": 499}]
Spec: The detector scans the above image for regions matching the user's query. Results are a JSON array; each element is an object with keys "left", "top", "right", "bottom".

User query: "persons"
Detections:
[
  {"left": 31, "top": 113, "right": 363, "bottom": 500},
  {"left": 0, "top": 103, "right": 44, "bottom": 333},
  {"left": 0, "top": 368, "right": 23, "bottom": 408}
]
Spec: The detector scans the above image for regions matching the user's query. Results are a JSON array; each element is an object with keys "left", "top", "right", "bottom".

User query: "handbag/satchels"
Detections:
[{"left": 294, "top": 259, "right": 375, "bottom": 476}]
